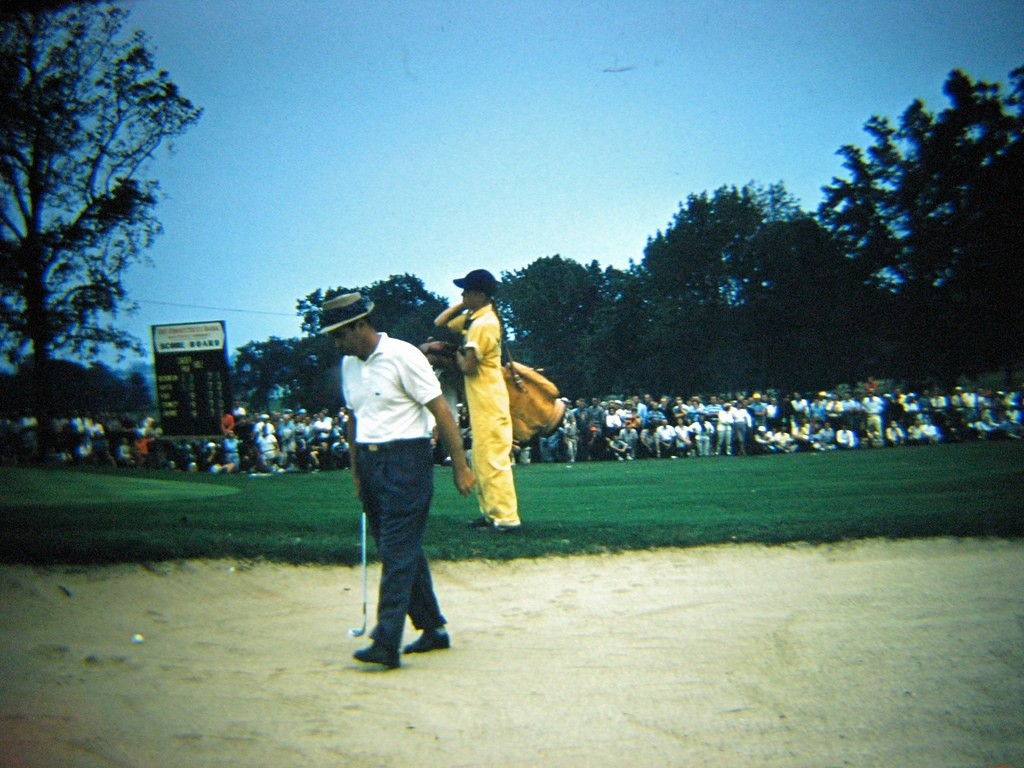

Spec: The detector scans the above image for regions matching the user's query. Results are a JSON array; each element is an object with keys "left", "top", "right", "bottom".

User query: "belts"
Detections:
[{"left": 355, "top": 438, "right": 430, "bottom": 451}]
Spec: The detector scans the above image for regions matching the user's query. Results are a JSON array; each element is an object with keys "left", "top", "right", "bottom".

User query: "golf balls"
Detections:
[
  {"left": 228, "top": 566, "right": 235, "bottom": 574},
  {"left": 130, "top": 633, "right": 144, "bottom": 644}
]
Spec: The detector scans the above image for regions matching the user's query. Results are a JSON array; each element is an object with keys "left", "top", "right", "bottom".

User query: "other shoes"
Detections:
[
  {"left": 468, "top": 516, "right": 493, "bottom": 531},
  {"left": 480, "top": 525, "right": 523, "bottom": 535}
]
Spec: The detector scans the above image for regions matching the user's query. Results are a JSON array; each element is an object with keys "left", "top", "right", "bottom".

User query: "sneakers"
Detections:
[
  {"left": 354, "top": 641, "right": 401, "bottom": 667},
  {"left": 403, "top": 628, "right": 450, "bottom": 654}
]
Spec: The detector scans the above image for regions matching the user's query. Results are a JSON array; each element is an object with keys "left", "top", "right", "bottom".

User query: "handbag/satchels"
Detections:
[{"left": 465, "top": 298, "right": 566, "bottom": 441}]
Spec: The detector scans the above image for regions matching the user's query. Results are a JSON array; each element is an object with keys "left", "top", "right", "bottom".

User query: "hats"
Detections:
[
  {"left": 453, "top": 270, "right": 498, "bottom": 296},
  {"left": 315, "top": 291, "right": 375, "bottom": 333}
]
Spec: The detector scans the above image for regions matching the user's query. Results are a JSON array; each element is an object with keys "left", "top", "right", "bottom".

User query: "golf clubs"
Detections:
[{"left": 348, "top": 512, "right": 368, "bottom": 638}]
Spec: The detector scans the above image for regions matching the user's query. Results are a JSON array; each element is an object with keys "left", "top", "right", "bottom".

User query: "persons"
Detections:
[
  {"left": 431, "top": 406, "right": 474, "bottom": 466},
  {"left": 1, "top": 405, "right": 353, "bottom": 477},
  {"left": 434, "top": 268, "right": 523, "bottom": 536},
  {"left": 512, "top": 386, "right": 1024, "bottom": 466},
  {"left": 322, "top": 290, "right": 476, "bottom": 669}
]
